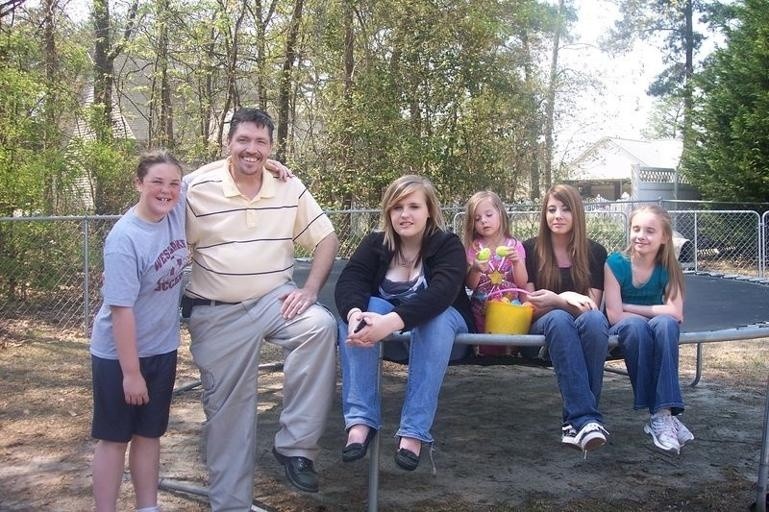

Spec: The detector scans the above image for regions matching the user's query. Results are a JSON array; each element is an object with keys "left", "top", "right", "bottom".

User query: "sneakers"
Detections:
[
  {"left": 643, "top": 414, "right": 694, "bottom": 456},
  {"left": 561, "top": 423, "right": 607, "bottom": 452}
]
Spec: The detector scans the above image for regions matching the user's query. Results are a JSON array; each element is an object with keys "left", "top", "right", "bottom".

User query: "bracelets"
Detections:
[{"left": 348, "top": 309, "right": 361, "bottom": 320}]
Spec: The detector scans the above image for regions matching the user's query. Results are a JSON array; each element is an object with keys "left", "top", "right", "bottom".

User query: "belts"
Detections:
[{"left": 192, "top": 298, "right": 223, "bottom": 306}]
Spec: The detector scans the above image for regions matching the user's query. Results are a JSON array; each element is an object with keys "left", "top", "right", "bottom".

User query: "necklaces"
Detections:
[
  {"left": 397, "top": 246, "right": 420, "bottom": 269},
  {"left": 630, "top": 266, "right": 651, "bottom": 288}
]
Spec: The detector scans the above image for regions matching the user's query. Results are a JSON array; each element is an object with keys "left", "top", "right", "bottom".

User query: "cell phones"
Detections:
[{"left": 354, "top": 318, "right": 367, "bottom": 333}]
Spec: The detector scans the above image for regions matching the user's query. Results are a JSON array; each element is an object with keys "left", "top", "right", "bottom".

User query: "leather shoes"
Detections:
[
  {"left": 271, "top": 447, "right": 319, "bottom": 493},
  {"left": 342, "top": 426, "right": 376, "bottom": 462},
  {"left": 395, "top": 436, "right": 422, "bottom": 472}
]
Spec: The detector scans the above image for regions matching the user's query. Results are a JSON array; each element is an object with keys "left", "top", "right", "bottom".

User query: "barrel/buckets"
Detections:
[{"left": 483, "top": 287, "right": 536, "bottom": 335}]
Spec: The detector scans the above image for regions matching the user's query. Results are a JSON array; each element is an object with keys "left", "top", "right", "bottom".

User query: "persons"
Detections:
[
  {"left": 86, "top": 150, "right": 295, "bottom": 511},
  {"left": 601, "top": 203, "right": 696, "bottom": 458},
  {"left": 516, "top": 183, "right": 609, "bottom": 454},
  {"left": 461, "top": 189, "right": 530, "bottom": 359},
  {"left": 180, "top": 107, "right": 341, "bottom": 511},
  {"left": 334, "top": 174, "right": 482, "bottom": 473}
]
